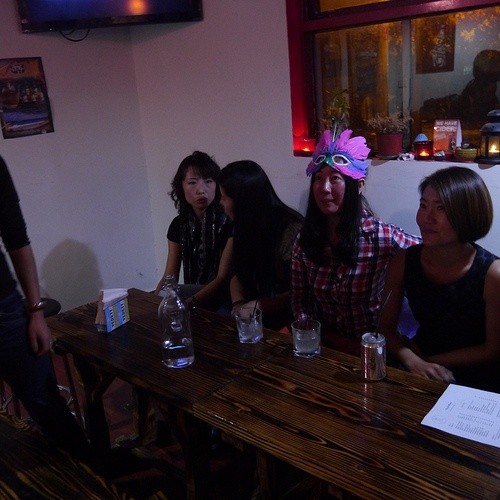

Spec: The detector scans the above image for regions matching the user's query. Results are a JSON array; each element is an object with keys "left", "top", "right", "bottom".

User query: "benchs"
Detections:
[{"left": 0, "top": 414, "right": 134, "bottom": 500}]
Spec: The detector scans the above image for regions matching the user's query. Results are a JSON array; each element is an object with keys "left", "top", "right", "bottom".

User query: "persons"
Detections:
[
  {"left": 278, "top": 121, "right": 422, "bottom": 356},
  {"left": 148, "top": 150, "right": 234, "bottom": 304},
  {"left": 447, "top": 49, "right": 500, "bottom": 129},
  {"left": 220, "top": 160, "right": 304, "bottom": 332},
  {"left": 376, "top": 166, "right": 500, "bottom": 382},
  {"left": 0, "top": 154, "right": 96, "bottom": 468}
]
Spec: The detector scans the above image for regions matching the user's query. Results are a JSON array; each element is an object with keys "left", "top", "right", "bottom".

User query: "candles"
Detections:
[{"left": 415, "top": 139, "right": 433, "bottom": 160}]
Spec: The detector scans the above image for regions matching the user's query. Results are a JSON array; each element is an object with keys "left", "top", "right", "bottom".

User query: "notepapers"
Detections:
[{"left": 95, "top": 289, "right": 128, "bottom": 325}]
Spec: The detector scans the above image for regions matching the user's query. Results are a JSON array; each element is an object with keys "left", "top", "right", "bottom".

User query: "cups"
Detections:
[
  {"left": 234, "top": 307, "right": 263, "bottom": 344},
  {"left": 413, "top": 141, "right": 433, "bottom": 161},
  {"left": 291, "top": 319, "right": 321, "bottom": 358}
]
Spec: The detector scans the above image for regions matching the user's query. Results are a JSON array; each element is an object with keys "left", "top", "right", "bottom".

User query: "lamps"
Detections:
[{"left": 473, "top": 108, "right": 500, "bottom": 166}]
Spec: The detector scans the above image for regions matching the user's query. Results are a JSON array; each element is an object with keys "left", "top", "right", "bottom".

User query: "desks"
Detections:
[{"left": 47, "top": 286, "right": 500, "bottom": 500}]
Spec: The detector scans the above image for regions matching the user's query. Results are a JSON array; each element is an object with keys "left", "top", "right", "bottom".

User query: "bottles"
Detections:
[{"left": 157, "top": 274, "right": 194, "bottom": 369}]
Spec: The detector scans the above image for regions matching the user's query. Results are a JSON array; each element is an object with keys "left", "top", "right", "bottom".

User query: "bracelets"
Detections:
[
  {"left": 28, "top": 301, "right": 47, "bottom": 311},
  {"left": 191, "top": 296, "right": 198, "bottom": 304},
  {"left": 232, "top": 300, "right": 246, "bottom": 306}
]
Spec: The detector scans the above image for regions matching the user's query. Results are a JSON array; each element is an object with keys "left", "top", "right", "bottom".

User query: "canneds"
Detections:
[{"left": 361, "top": 332, "right": 386, "bottom": 381}]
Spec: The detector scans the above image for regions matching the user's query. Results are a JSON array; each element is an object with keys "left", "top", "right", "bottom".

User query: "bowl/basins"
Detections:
[{"left": 455, "top": 148, "right": 477, "bottom": 163}]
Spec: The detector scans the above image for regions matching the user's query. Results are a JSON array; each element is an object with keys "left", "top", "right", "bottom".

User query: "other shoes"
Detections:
[{"left": 129, "top": 404, "right": 168, "bottom": 459}]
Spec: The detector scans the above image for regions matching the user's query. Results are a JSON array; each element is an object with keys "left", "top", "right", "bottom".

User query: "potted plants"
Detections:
[{"left": 363, "top": 107, "right": 417, "bottom": 158}]
